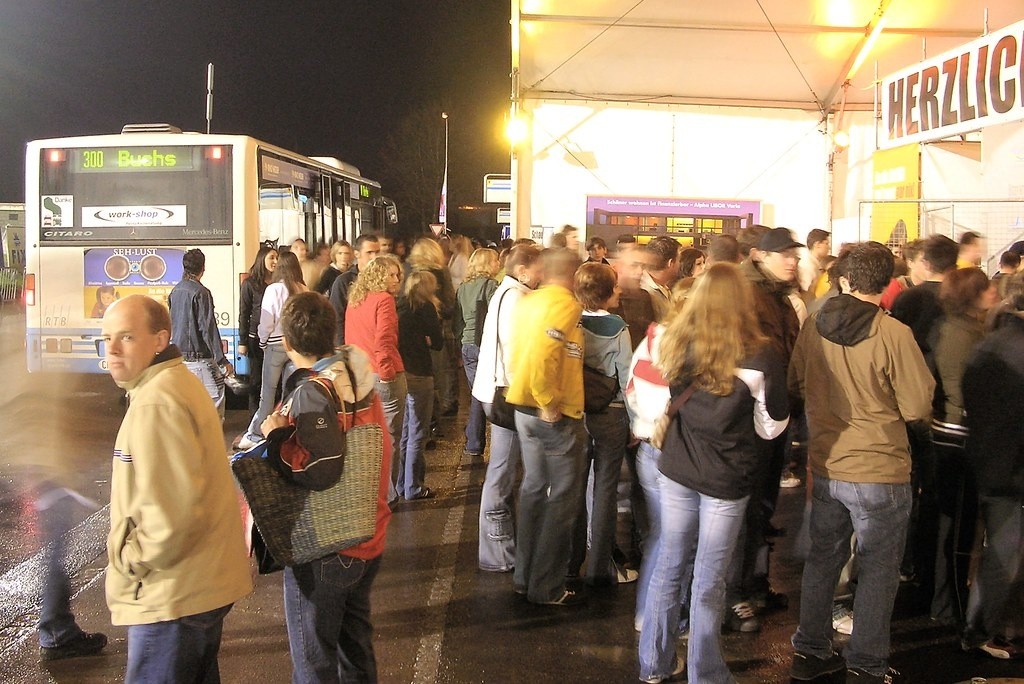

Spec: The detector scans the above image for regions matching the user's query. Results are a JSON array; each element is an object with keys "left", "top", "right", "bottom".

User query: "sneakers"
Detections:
[
  {"left": 789, "top": 648, "right": 845, "bottom": 681},
  {"left": 847, "top": 665, "right": 909, "bottom": 684},
  {"left": 748, "top": 575, "right": 789, "bottom": 611},
  {"left": 725, "top": 592, "right": 762, "bottom": 632}
]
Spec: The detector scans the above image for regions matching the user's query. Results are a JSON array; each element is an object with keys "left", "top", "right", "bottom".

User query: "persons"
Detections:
[
  {"left": 99, "top": 295, "right": 254, "bottom": 684},
  {"left": 637, "top": 264, "right": 791, "bottom": 684},
  {"left": 169, "top": 249, "right": 233, "bottom": 426},
  {"left": 236, "top": 233, "right": 474, "bottom": 511},
  {"left": 789, "top": 241, "right": 936, "bottom": 684},
  {"left": 92, "top": 286, "right": 120, "bottom": 318},
  {"left": 260, "top": 292, "right": 392, "bottom": 684},
  {"left": 454, "top": 224, "right": 1023, "bottom": 661}
]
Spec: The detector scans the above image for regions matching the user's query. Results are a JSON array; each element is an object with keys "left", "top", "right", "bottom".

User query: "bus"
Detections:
[{"left": 25, "top": 123, "right": 399, "bottom": 382}]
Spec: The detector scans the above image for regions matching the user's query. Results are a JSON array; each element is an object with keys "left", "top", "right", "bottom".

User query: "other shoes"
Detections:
[
  {"left": 39, "top": 631, "right": 109, "bottom": 662},
  {"left": 980, "top": 635, "right": 1024, "bottom": 659},
  {"left": 414, "top": 488, "right": 436, "bottom": 500},
  {"left": 779, "top": 477, "right": 800, "bottom": 487},
  {"left": 678, "top": 624, "right": 689, "bottom": 640},
  {"left": 832, "top": 594, "right": 854, "bottom": 635},
  {"left": 537, "top": 588, "right": 582, "bottom": 606},
  {"left": 616, "top": 567, "right": 639, "bottom": 583},
  {"left": 643, "top": 656, "right": 685, "bottom": 684},
  {"left": 462, "top": 447, "right": 480, "bottom": 456},
  {"left": 766, "top": 526, "right": 783, "bottom": 537}
]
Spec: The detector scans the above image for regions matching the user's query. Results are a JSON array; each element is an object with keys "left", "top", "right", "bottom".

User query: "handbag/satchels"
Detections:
[
  {"left": 583, "top": 366, "right": 619, "bottom": 416},
  {"left": 248, "top": 524, "right": 280, "bottom": 575},
  {"left": 474, "top": 279, "right": 489, "bottom": 349},
  {"left": 231, "top": 376, "right": 383, "bottom": 568},
  {"left": 489, "top": 386, "right": 517, "bottom": 431},
  {"left": 651, "top": 398, "right": 674, "bottom": 451}
]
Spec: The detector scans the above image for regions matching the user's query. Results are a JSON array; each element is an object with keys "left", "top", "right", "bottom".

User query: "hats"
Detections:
[{"left": 758, "top": 228, "right": 806, "bottom": 253}]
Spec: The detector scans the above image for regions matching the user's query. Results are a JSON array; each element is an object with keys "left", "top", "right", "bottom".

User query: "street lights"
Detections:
[{"left": 441, "top": 112, "right": 450, "bottom": 235}]
